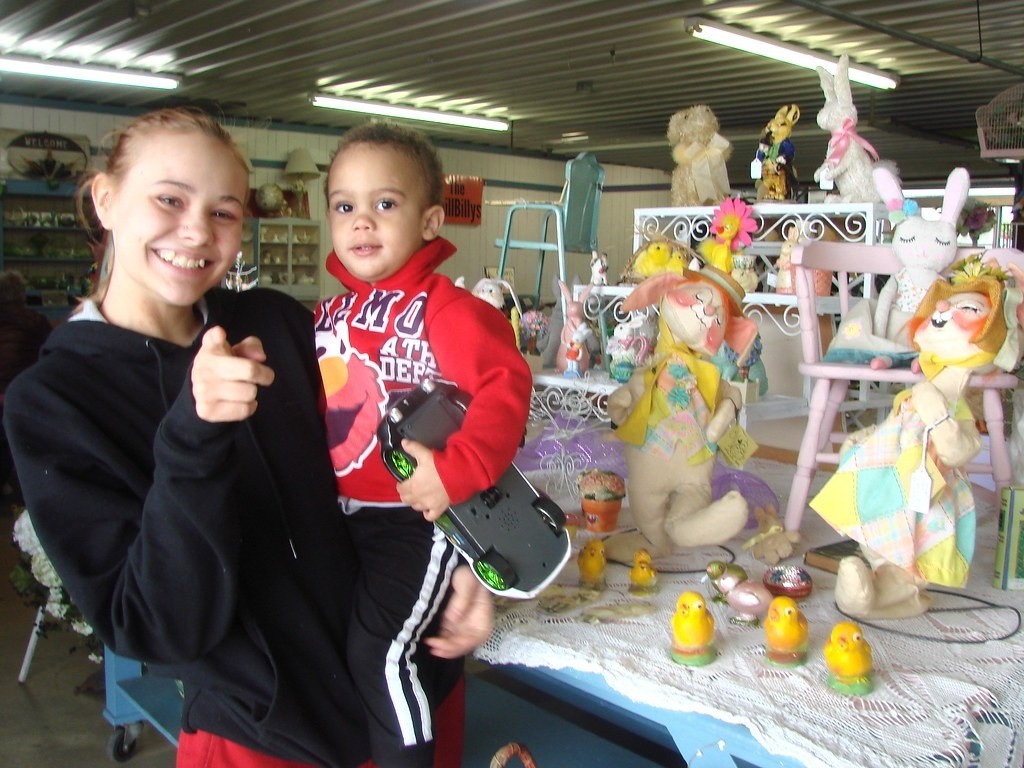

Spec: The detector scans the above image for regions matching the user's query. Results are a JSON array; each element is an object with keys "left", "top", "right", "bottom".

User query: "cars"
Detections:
[{"left": 376, "top": 376, "right": 571, "bottom": 600}]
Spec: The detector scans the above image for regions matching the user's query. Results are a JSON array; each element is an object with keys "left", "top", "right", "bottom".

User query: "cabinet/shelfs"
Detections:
[
  {"left": 0, "top": 177, "right": 104, "bottom": 321},
  {"left": 221, "top": 217, "right": 323, "bottom": 303},
  {"left": 529, "top": 201, "right": 888, "bottom": 469}
]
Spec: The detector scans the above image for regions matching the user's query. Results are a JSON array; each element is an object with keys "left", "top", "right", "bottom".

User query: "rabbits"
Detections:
[{"left": 553, "top": 280, "right": 594, "bottom": 375}]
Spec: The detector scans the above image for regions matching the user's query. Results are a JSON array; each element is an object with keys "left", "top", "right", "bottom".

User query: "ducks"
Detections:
[{"left": 578, "top": 540, "right": 874, "bottom": 695}]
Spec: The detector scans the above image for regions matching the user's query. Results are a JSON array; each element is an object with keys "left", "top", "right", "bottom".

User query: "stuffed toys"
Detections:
[
  {"left": 753, "top": 503, "right": 800, "bottom": 562},
  {"left": 472, "top": 278, "right": 523, "bottom": 318},
  {"left": 814, "top": 56, "right": 896, "bottom": 204},
  {"left": 806, "top": 257, "right": 1023, "bottom": 613},
  {"left": 634, "top": 241, "right": 688, "bottom": 275},
  {"left": 666, "top": 104, "right": 731, "bottom": 205},
  {"left": 868, "top": 166, "right": 970, "bottom": 372},
  {"left": 604, "top": 264, "right": 757, "bottom": 561}
]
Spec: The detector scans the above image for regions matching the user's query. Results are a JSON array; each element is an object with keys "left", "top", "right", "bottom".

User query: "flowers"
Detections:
[
  {"left": 6, "top": 508, "right": 105, "bottom": 665},
  {"left": 575, "top": 468, "right": 627, "bottom": 501}
]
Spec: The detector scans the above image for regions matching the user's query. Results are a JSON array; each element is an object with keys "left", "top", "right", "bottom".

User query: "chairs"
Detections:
[
  {"left": 493, "top": 151, "right": 611, "bottom": 372},
  {"left": 780, "top": 238, "right": 1024, "bottom": 530}
]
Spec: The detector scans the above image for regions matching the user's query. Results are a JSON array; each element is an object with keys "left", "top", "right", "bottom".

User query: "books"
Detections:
[
  {"left": 804, "top": 540, "right": 871, "bottom": 575},
  {"left": 994, "top": 486, "right": 1024, "bottom": 590}
]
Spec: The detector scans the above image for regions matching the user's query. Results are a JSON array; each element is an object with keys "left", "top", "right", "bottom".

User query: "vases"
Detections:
[{"left": 582, "top": 498, "right": 622, "bottom": 533}]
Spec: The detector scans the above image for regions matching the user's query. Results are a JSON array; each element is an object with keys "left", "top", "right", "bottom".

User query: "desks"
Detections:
[{"left": 98, "top": 420, "right": 1024, "bottom": 768}]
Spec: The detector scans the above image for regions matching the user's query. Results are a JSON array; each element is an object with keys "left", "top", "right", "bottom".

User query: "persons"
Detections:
[
  {"left": 0, "top": 269, "right": 53, "bottom": 506},
  {"left": 0, "top": 108, "right": 492, "bottom": 767},
  {"left": 314, "top": 121, "right": 533, "bottom": 767}
]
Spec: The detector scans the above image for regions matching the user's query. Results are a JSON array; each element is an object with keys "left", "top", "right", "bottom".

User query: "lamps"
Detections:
[
  {"left": 683, "top": 16, "right": 902, "bottom": 92},
  {"left": 282, "top": 147, "right": 321, "bottom": 218},
  {"left": 900, "top": 179, "right": 1016, "bottom": 199},
  {"left": 0, "top": 53, "right": 184, "bottom": 90},
  {"left": 307, "top": 91, "right": 511, "bottom": 134}
]
